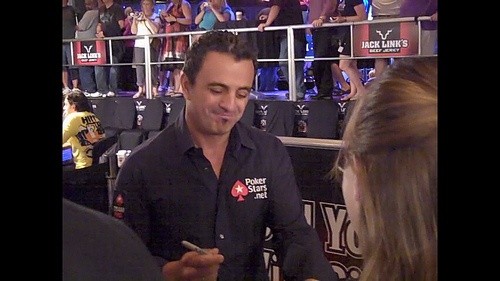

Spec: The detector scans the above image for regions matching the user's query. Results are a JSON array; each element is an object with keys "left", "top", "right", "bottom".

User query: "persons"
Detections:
[{"left": 63, "top": 0, "right": 437, "bottom": 281}]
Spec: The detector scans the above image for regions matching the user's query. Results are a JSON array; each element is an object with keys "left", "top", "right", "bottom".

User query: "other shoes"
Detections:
[
  {"left": 309, "top": 93, "right": 333, "bottom": 100},
  {"left": 340, "top": 94, "right": 358, "bottom": 101}
]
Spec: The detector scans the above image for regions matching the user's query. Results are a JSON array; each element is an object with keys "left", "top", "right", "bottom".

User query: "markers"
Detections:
[{"left": 182, "top": 240, "right": 209, "bottom": 256}]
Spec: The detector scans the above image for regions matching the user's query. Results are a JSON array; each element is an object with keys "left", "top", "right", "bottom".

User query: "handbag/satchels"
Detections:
[{"left": 150, "top": 38, "right": 161, "bottom": 52}]
